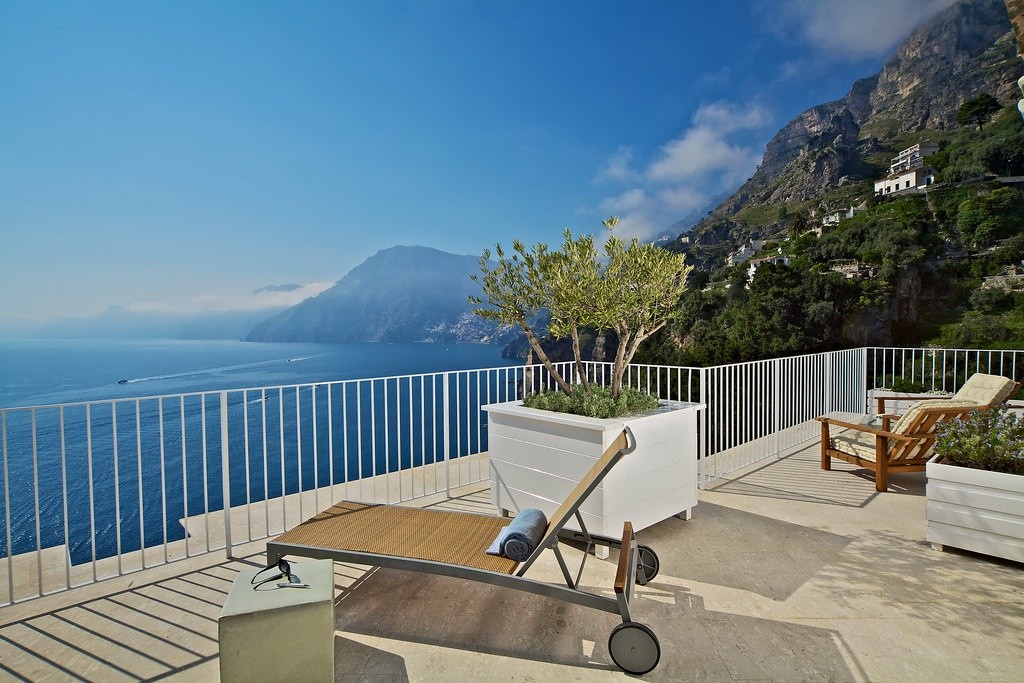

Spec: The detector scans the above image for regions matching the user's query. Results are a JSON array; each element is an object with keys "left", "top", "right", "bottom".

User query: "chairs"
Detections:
[
  {"left": 815, "top": 372, "right": 1024, "bottom": 493},
  {"left": 266, "top": 426, "right": 662, "bottom": 676}
]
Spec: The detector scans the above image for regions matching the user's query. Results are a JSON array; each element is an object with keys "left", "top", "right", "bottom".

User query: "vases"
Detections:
[{"left": 924, "top": 453, "right": 1024, "bottom": 565}]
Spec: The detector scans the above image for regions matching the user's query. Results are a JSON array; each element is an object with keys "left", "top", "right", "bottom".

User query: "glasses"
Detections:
[{"left": 251, "top": 559, "right": 291, "bottom": 590}]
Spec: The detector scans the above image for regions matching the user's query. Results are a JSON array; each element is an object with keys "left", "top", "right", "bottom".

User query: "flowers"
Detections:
[{"left": 932, "top": 402, "right": 1024, "bottom": 474}]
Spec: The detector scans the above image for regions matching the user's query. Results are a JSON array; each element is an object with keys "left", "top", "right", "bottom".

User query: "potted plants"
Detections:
[{"left": 467, "top": 214, "right": 707, "bottom": 560}]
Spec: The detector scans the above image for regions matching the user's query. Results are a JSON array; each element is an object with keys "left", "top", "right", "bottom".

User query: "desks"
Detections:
[{"left": 219, "top": 558, "right": 337, "bottom": 683}]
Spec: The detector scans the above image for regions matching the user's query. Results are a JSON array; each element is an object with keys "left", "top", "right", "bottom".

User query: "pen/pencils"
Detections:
[{"left": 277, "top": 582, "right": 310, "bottom": 588}]
